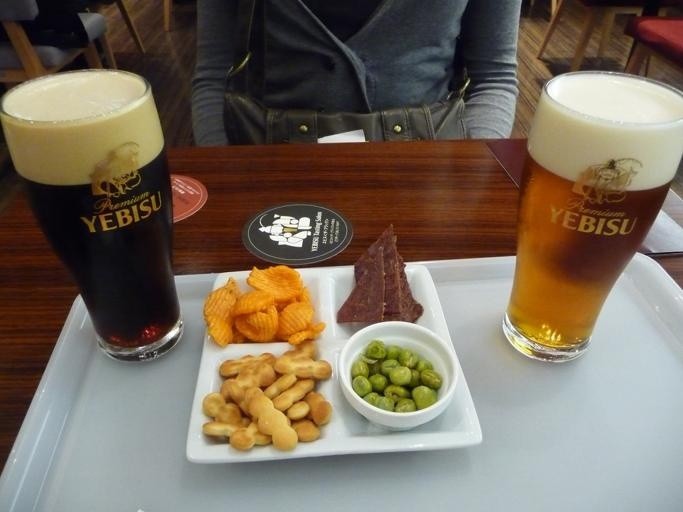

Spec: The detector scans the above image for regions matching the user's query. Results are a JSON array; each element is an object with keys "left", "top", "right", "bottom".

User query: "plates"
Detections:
[{"left": 185, "top": 261, "right": 485, "bottom": 465}]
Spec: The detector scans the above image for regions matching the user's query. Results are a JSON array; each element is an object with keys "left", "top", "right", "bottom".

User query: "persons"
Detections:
[{"left": 188, "top": 0, "right": 522, "bottom": 147}]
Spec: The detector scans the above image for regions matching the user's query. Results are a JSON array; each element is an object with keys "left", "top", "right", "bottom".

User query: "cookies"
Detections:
[{"left": 202, "top": 339, "right": 332, "bottom": 449}]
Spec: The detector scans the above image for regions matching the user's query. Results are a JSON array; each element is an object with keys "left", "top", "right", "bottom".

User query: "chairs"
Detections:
[{"left": 0, "top": 17, "right": 102, "bottom": 84}]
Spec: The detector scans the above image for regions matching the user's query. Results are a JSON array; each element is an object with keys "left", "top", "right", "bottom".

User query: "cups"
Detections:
[
  {"left": 0, "top": 67, "right": 185, "bottom": 365},
  {"left": 501, "top": 71, "right": 682, "bottom": 362}
]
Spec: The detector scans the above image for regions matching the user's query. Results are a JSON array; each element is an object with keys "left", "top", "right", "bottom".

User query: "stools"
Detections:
[{"left": 620, "top": 11, "right": 683, "bottom": 79}]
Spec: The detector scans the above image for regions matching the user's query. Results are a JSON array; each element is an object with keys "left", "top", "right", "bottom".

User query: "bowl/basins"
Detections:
[{"left": 335, "top": 320, "right": 461, "bottom": 430}]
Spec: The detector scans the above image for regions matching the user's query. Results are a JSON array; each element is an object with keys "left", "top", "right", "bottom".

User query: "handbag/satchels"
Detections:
[{"left": 222, "top": 93, "right": 466, "bottom": 210}]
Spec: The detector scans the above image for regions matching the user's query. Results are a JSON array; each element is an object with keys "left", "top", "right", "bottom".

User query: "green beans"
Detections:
[{"left": 352, "top": 340, "right": 441, "bottom": 412}]
